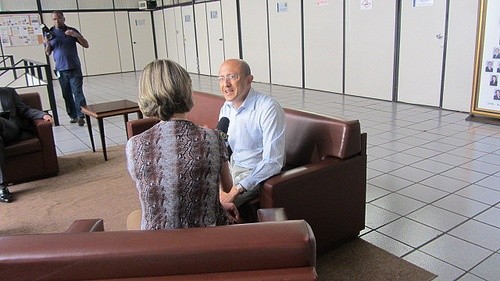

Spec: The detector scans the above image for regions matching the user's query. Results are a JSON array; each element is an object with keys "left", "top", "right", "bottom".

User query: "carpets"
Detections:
[{"left": 1, "top": 144, "right": 437, "bottom": 280}]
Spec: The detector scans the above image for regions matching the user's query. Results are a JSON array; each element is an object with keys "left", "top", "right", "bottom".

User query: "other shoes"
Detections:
[
  {"left": 70, "top": 118, "right": 78, "bottom": 123},
  {"left": 78, "top": 118, "right": 85, "bottom": 126}
]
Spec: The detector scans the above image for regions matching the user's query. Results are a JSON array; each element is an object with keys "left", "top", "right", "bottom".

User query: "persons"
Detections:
[
  {"left": 493, "top": 90, "right": 500, "bottom": 100},
  {"left": 0, "top": 87, "right": 55, "bottom": 203},
  {"left": 125, "top": 59, "right": 238, "bottom": 230},
  {"left": 43, "top": 11, "right": 89, "bottom": 126},
  {"left": 485, "top": 62, "right": 493, "bottom": 72},
  {"left": 490, "top": 75, "right": 497, "bottom": 86},
  {"left": 493, "top": 48, "right": 500, "bottom": 59},
  {"left": 218, "top": 58, "right": 287, "bottom": 222}
]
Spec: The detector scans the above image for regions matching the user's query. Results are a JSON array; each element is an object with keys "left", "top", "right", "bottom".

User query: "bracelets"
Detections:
[{"left": 237, "top": 184, "right": 244, "bottom": 194}]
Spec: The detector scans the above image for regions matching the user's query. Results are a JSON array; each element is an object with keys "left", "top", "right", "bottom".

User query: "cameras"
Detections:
[{"left": 40, "top": 24, "right": 56, "bottom": 40}]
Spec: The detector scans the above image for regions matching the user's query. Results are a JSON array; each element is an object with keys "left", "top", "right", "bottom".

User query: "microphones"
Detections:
[{"left": 217, "top": 117, "right": 230, "bottom": 133}]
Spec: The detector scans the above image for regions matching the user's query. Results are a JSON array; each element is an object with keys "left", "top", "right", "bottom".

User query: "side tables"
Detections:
[{"left": 80, "top": 99, "right": 143, "bottom": 161}]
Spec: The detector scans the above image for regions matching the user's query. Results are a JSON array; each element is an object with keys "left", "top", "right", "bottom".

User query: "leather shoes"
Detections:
[{"left": 0, "top": 187, "right": 13, "bottom": 202}]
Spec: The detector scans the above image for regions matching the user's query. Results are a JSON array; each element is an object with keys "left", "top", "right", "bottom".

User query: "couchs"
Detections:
[
  {"left": 0, "top": 92, "right": 60, "bottom": 186},
  {"left": 0, "top": 217, "right": 320, "bottom": 281},
  {"left": 126, "top": 91, "right": 369, "bottom": 257}
]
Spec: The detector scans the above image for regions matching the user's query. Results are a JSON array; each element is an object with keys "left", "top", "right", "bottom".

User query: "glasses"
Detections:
[{"left": 217, "top": 72, "right": 249, "bottom": 83}]
